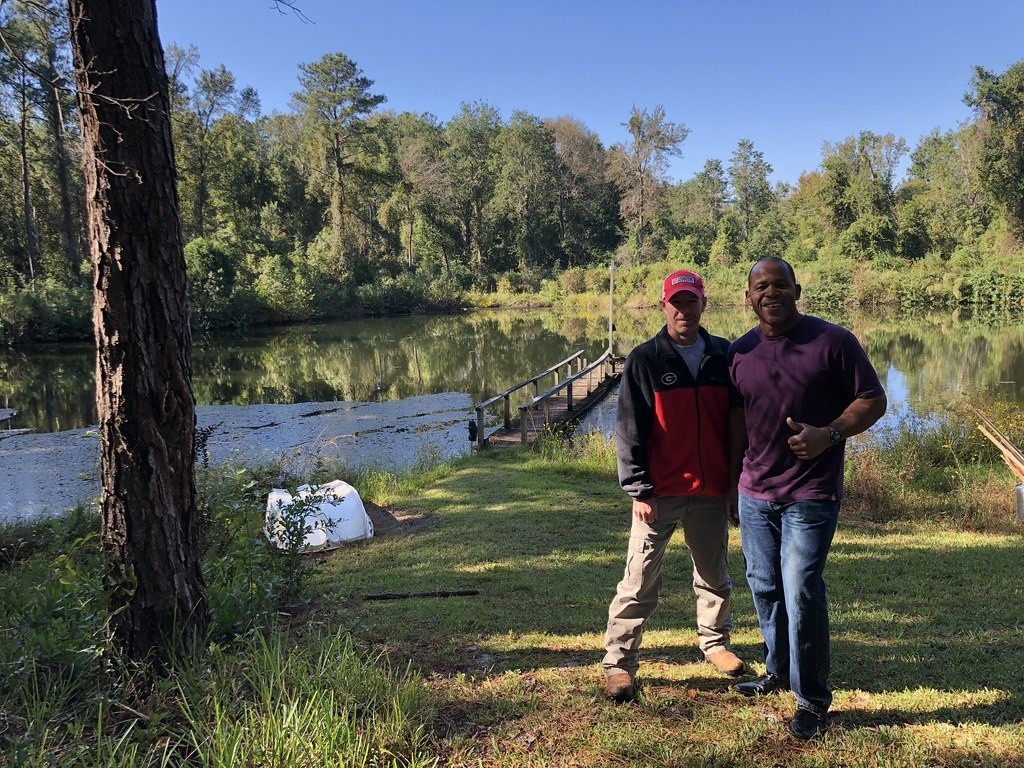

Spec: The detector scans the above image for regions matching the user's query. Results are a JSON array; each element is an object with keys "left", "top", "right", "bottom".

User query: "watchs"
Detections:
[{"left": 827, "top": 423, "right": 841, "bottom": 447}]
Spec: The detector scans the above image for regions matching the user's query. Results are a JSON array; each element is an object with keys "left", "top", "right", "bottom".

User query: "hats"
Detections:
[{"left": 662, "top": 269, "right": 704, "bottom": 303}]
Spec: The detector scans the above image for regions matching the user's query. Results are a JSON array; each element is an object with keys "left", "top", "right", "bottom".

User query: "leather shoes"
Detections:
[
  {"left": 732, "top": 673, "right": 790, "bottom": 696},
  {"left": 790, "top": 707, "right": 823, "bottom": 740}
]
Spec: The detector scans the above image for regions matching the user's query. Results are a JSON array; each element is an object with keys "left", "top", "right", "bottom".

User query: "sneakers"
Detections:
[
  {"left": 705, "top": 649, "right": 745, "bottom": 675},
  {"left": 606, "top": 673, "right": 635, "bottom": 700}
]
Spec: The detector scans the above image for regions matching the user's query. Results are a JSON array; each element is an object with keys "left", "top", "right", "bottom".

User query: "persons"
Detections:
[
  {"left": 601, "top": 270, "right": 745, "bottom": 697},
  {"left": 724, "top": 256, "right": 887, "bottom": 739}
]
